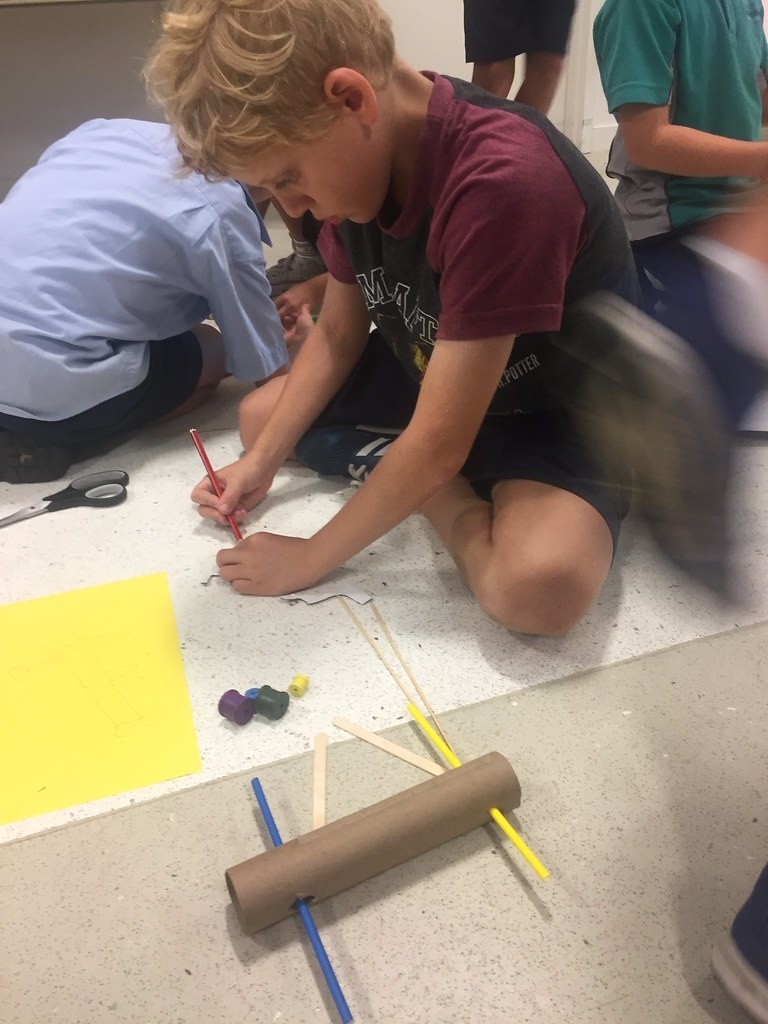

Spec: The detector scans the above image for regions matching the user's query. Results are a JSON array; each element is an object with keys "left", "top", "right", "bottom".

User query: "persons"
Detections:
[
  {"left": 712, "top": 864, "right": 768, "bottom": 1024},
  {"left": 0, "top": 0, "right": 768, "bottom": 637}
]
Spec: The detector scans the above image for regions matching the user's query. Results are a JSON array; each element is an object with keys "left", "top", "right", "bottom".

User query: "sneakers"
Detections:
[
  {"left": 294, "top": 423, "right": 409, "bottom": 488},
  {"left": 264, "top": 252, "right": 329, "bottom": 298}
]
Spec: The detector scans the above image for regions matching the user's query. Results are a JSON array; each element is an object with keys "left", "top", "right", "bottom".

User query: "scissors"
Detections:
[{"left": 0, "top": 470, "right": 128, "bottom": 530}]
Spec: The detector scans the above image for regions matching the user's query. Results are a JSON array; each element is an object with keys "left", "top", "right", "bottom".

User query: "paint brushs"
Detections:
[{"left": 190, "top": 428, "right": 243, "bottom": 543}]
